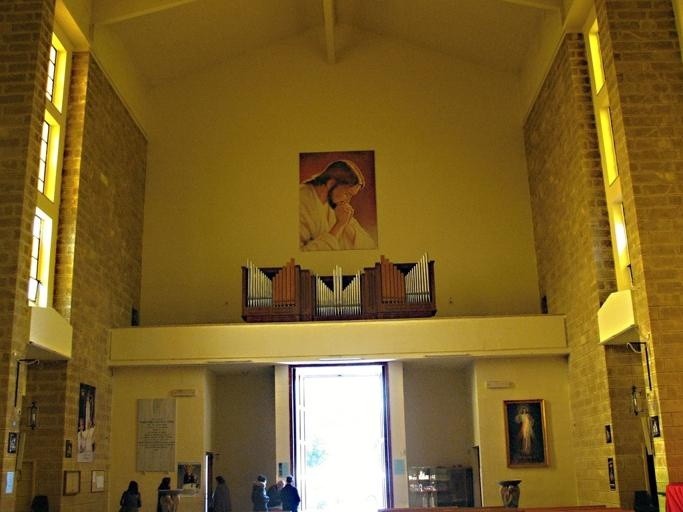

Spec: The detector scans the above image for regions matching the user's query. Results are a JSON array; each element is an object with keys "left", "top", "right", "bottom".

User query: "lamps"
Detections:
[
  {"left": 626, "top": 341, "right": 652, "bottom": 416},
  {"left": 13, "top": 358, "right": 40, "bottom": 430}
]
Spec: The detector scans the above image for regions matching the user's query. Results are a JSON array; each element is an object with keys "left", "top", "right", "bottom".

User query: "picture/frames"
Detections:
[
  {"left": 62, "top": 469, "right": 104, "bottom": 496},
  {"left": 502, "top": 398, "right": 549, "bottom": 468},
  {"left": 7, "top": 432, "right": 17, "bottom": 453}
]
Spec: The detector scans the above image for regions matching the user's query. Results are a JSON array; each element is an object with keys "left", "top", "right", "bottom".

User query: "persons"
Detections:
[
  {"left": 267, "top": 480, "right": 284, "bottom": 512},
  {"left": 297, "top": 158, "right": 374, "bottom": 250},
  {"left": 279, "top": 475, "right": 300, "bottom": 511},
  {"left": 250, "top": 474, "right": 270, "bottom": 512},
  {"left": 29, "top": 495, "right": 49, "bottom": 511},
  {"left": 210, "top": 475, "right": 231, "bottom": 511},
  {"left": 155, "top": 477, "right": 171, "bottom": 512},
  {"left": 513, "top": 404, "right": 536, "bottom": 459},
  {"left": 116, "top": 479, "right": 141, "bottom": 511}
]
